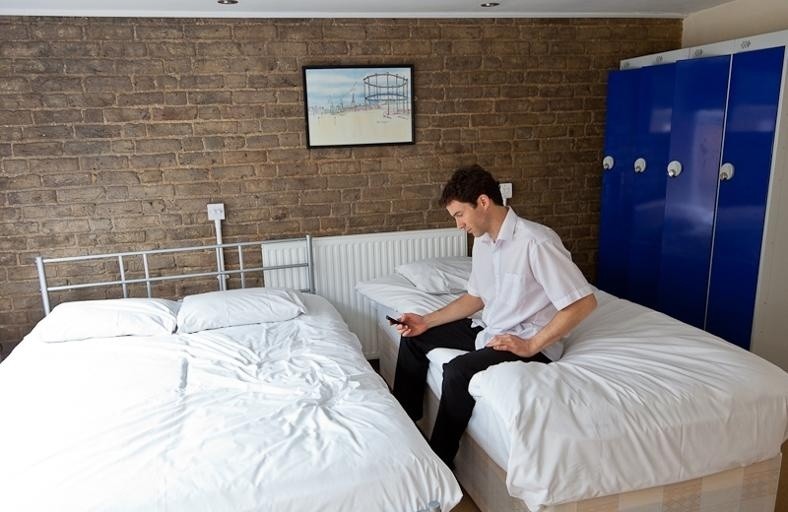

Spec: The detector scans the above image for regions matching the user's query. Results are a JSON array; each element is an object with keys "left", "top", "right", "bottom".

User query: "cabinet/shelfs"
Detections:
[{"left": 595, "top": 29, "right": 788, "bottom": 371}]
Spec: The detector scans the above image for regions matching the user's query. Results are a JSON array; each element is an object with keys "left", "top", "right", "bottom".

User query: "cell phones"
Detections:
[{"left": 386, "top": 316, "right": 402, "bottom": 324}]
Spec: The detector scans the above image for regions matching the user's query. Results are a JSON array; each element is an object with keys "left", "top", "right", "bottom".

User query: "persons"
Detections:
[{"left": 390, "top": 166, "right": 600, "bottom": 469}]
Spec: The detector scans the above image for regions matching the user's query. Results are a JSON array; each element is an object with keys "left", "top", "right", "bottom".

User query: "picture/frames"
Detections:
[{"left": 302, "top": 64, "right": 415, "bottom": 150}]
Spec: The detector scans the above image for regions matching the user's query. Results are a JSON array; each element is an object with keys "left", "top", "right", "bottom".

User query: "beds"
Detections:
[
  {"left": 379, "top": 285, "right": 788, "bottom": 512},
  {"left": 0, "top": 235, "right": 463, "bottom": 512}
]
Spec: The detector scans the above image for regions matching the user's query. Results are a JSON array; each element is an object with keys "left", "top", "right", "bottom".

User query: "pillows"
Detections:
[
  {"left": 176, "top": 287, "right": 308, "bottom": 336},
  {"left": 394, "top": 256, "right": 472, "bottom": 294},
  {"left": 41, "top": 298, "right": 181, "bottom": 343}
]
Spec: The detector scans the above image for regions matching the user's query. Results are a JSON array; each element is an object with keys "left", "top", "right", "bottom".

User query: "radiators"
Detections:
[{"left": 262, "top": 228, "right": 468, "bottom": 360}]
